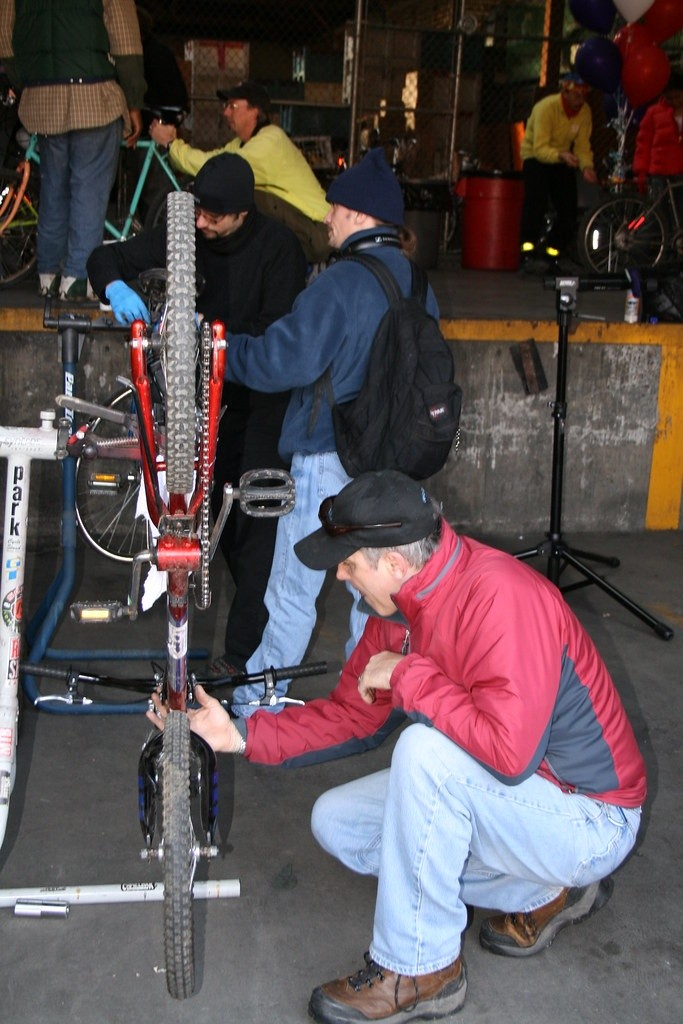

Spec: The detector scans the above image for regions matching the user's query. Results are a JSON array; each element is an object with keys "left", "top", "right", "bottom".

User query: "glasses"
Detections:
[
  {"left": 195, "top": 207, "right": 228, "bottom": 224},
  {"left": 318, "top": 495, "right": 402, "bottom": 538},
  {"left": 223, "top": 102, "right": 254, "bottom": 112}
]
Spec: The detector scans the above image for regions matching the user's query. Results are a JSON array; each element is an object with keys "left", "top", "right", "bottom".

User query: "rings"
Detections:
[{"left": 357, "top": 675, "right": 362, "bottom": 682}]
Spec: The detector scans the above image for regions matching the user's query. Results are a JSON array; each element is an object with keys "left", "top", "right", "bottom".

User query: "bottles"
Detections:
[
  {"left": 650, "top": 317, "right": 657, "bottom": 323},
  {"left": 462, "top": 152, "right": 470, "bottom": 170}
]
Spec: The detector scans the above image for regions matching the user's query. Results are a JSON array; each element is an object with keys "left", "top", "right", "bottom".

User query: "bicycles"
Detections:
[
  {"left": 0, "top": 102, "right": 218, "bottom": 291},
  {"left": 14, "top": 188, "right": 329, "bottom": 999},
  {"left": 577, "top": 176, "right": 683, "bottom": 277}
]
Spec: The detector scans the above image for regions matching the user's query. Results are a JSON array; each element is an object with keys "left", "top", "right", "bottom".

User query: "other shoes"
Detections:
[
  {"left": 524, "top": 258, "right": 550, "bottom": 272},
  {"left": 555, "top": 258, "right": 571, "bottom": 275}
]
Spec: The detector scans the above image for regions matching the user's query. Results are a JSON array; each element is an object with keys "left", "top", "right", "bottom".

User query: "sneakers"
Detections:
[
  {"left": 308, "top": 951, "right": 468, "bottom": 1024},
  {"left": 36, "top": 272, "right": 62, "bottom": 298},
  {"left": 59, "top": 275, "right": 100, "bottom": 303},
  {"left": 190, "top": 657, "right": 244, "bottom": 680},
  {"left": 479, "top": 876, "right": 613, "bottom": 957}
]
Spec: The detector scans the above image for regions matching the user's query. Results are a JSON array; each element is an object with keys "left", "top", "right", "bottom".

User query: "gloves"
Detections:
[
  {"left": 105, "top": 280, "right": 151, "bottom": 328},
  {"left": 153, "top": 312, "right": 200, "bottom": 337}
]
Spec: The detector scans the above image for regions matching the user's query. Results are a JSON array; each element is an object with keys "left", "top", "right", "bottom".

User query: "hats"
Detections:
[
  {"left": 216, "top": 82, "right": 271, "bottom": 112},
  {"left": 293, "top": 470, "right": 440, "bottom": 570},
  {"left": 325, "top": 147, "right": 403, "bottom": 226},
  {"left": 192, "top": 151, "right": 255, "bottom": 213}
]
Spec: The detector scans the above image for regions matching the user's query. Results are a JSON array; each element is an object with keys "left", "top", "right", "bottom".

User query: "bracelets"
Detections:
[
  {"left": 238, "top": 735, "right": 246, "bottom": 754},
  {"left": 167, "top": 140, "right": 176, "bottom": 152}
]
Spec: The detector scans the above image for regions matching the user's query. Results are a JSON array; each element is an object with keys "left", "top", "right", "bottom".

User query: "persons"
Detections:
[
  {"left": 622, "top": 66, "right": 683, "bottom": 324},
  {"left": 475, "top": 147, "right": 514, "bottom": 178},
  {"left": 222, "top": 146, "right": 440, "bottom": 719},
  {"left": 86, "top": 151, "right": 306, "bottom": 679},
  {"left": 148, "top": 80, "right": 335, "bottom": 266},
  {"left": 518, "top": 74, "right": 597, "bottom": 279},
  {"left": 146, "top": 471, "right": 648, "bottom": 1024},
  {"left": 0, "top": 0, "right": 147, "bottom": 307}
]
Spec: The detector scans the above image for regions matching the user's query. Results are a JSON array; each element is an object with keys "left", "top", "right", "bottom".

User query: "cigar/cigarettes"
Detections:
[{"left": 159, "top": 118, "right": 163, "bottom": 124}]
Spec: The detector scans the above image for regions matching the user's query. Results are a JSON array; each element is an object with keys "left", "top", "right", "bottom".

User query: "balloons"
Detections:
[
  {"left": 613, "top": 0, "right": 654, "bottom": 25},
  {"left": 641, "top": 0, "right": 683, "bottom": 43},
  {"left": 621, "top": 45, "right": 671, "bottom": 109},
  {"left": 568, "top": 0, "right": 617, "bottom": 37},
  {"left": 613, "top": 24, "right": 657, "bottom": 57},
  {"left": 576, "top": 34, "right": 624, "bottom": 96}
]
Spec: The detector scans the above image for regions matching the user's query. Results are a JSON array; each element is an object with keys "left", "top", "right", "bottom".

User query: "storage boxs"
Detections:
[{"left": 170, "top": 1, "right": 488, "bottom": 176}]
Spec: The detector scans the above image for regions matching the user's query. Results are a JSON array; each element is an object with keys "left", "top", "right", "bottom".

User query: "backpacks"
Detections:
[{"left": 306, "top": 249, "right": 463, "bottom": 481}]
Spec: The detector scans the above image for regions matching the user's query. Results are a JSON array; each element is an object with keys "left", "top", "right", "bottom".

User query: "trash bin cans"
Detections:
[{"left": 454, "top": 168, "right": 527, "bottom": 272}]
